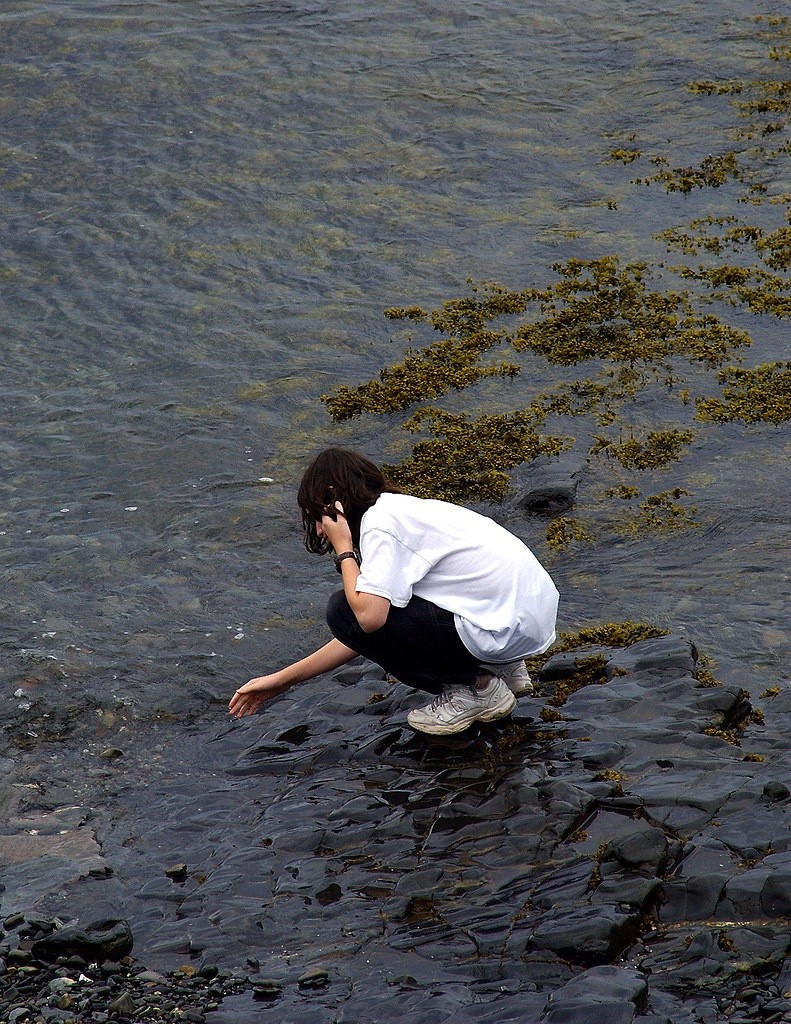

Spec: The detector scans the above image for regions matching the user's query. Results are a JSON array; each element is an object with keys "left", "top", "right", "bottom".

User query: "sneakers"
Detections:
[
  {"left": 407, "top": 675, "right": 517, "bottom": 735},
  {"left": 480, "top": 658, "right": 535, "bottom": 699}
]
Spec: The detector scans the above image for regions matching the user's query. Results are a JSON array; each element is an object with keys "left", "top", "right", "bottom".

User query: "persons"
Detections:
[{"left": 228, "top": 447, "right": 559, "bottom": 734}]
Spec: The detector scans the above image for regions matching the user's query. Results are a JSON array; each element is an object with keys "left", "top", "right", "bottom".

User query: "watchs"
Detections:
[{"left": 333, "top": 551, "right": 359, "bottom": 574}]
